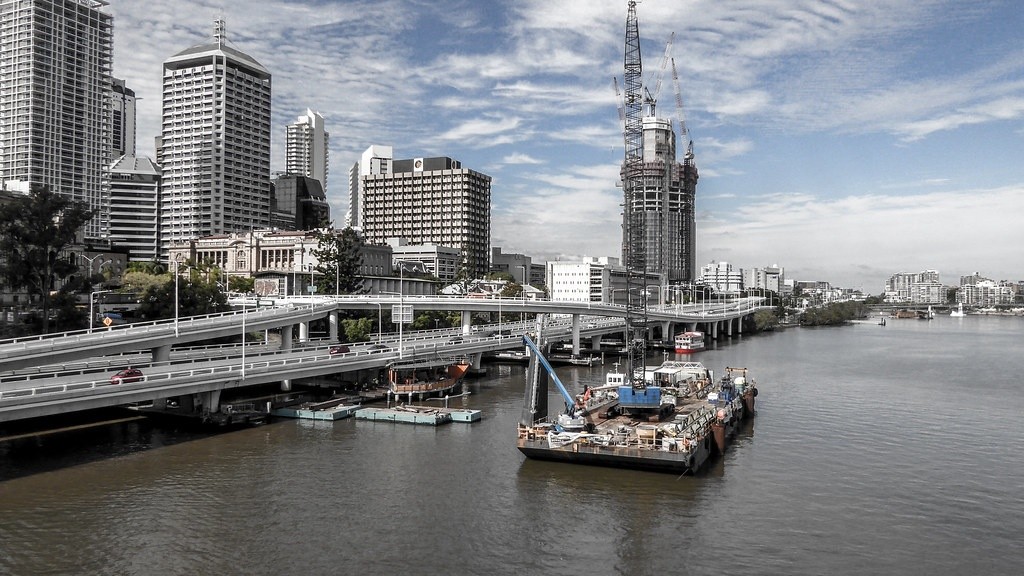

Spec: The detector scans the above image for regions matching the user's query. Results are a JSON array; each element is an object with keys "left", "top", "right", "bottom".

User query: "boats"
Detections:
[
  {"left": 675, "top": 332, "right": 705, "bottom": 353},
  {"left": 518, "top": 333, "right": 759, "bottom": 471}
]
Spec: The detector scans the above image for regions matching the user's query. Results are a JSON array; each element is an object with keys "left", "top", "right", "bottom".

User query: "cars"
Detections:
[
  {"left": 110, "top": 370, "right": 144, "bottom": 385},
  {"left": 329, "top": 345, "right": 350, "bottom": 354},
  {"left": 449, "top": 337, "right": 463, "bottom": 344},
  {"left": 367, "top": 345, "right": 390, "bottom": 354},
  {"left": 489, "top": 330, "right": 511, "bottom": 339}
]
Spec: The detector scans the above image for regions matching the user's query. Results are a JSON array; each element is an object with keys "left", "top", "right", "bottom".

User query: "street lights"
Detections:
[
  {"left": 169, "top": 260, "right": 179, "bottom": 338},
  {"left": 79, "top": 254, "right": 103, "bottom": 278},
  {"left": 333, "top": 264, "right": 339, "bottom": 308},
  {"left": 309, "top": 265, "right": 314, "bottom": 316},
  {"left": 99, "top": 260, "right": 111, "bottom": 274},
  {"left": 492, "top": 292, "right": 501, "bottom": 346},
  {"left": 90, "top": 291, "right": 112, "bottom": 334},
  {"left": 517, "top": 266, "right": 525, "bottom": 306}
]
